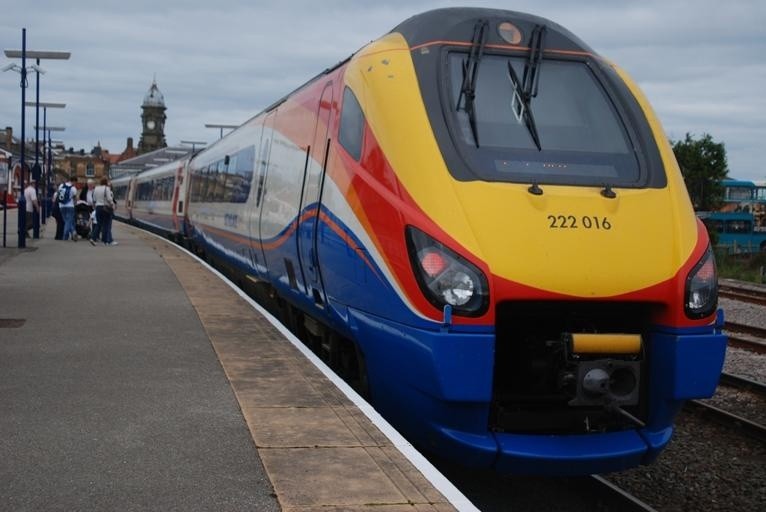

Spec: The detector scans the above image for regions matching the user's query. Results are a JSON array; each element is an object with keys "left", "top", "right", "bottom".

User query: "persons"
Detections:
[{"left": 24, "top": 177, "right": 119, "bottom": 247}]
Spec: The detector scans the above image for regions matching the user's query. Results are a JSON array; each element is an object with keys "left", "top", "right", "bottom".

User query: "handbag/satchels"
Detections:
[{"left": 102, "top": 206, "right": 112, "bottom": 216}]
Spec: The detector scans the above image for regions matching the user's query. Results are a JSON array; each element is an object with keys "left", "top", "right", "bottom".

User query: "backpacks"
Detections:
[{"left": 57, "top": 182, "right": 75, "bottom": 204}]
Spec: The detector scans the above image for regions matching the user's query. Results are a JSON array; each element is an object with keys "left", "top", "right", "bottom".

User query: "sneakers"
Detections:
[{"left": 73, "top": 230, "right": 119, "bottom": 245}]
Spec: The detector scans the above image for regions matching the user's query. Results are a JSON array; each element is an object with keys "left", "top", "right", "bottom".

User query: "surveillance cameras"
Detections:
[
  {"left": 2, "top": 62, "right": 17, "bottom": 72},
  {"left": 32, "top": 64, "right": 47, "bottom": 75}
]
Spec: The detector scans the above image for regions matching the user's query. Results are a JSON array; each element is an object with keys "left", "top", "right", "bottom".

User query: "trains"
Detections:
[{"left": 108, "top": 8, "right": 729, "bottom": 478}]
